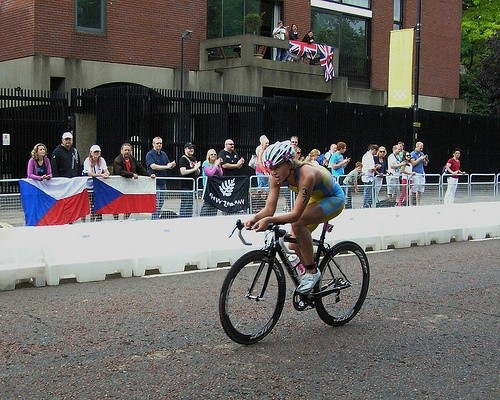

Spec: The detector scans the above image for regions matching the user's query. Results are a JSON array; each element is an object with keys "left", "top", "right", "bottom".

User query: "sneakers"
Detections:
[{"left": 296, "top": 268, "right": 322, "bottom": 294}]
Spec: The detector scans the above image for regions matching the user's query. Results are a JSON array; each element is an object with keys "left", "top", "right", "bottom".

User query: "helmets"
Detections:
[{"left": 262, "top": 140, "right": 296, "bottom": 170}]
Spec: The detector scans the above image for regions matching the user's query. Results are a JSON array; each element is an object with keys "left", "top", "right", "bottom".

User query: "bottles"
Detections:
[{"left": 288, "top": 254, "right": 305, "bottom": 276}]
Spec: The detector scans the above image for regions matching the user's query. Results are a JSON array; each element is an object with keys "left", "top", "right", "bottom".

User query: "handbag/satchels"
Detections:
[
  {"left": 442, "top": 166, "right": 448, "bottom": 174},
  {"left": 249, "top": 155, "right": 257, "bottom": 170}
]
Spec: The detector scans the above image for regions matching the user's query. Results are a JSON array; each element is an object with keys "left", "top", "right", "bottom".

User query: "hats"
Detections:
[
  {"left": 90, "top": 145, "right": 101, "bottom": 152},
  {"left": 185, "top": 143, "right": 196, "bottom": 149},
  {"left": 62, "top": 132, "right": 73, "bottom": 139}
]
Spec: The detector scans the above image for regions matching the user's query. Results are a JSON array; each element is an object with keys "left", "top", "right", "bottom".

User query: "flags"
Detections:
[{"left": 286, "top": 40, "right": 335, "bottom": 82}]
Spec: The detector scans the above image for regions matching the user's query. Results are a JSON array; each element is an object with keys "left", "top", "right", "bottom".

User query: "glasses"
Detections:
[
  {"left": 380, "top": 151, "right": 386, "bottom": 153},
  {"left": 405, "top": 154, "right": 412, "bottom": 157}
]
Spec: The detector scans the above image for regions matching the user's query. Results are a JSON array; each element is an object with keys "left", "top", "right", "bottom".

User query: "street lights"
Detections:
[{"left": 178, "top": 30, "right": 194, "bottom": 156}]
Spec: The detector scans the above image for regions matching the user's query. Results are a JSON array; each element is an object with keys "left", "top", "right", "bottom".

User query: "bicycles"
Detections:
[{"left": 217, "top": 219, "right": 371, "bottom": 345}]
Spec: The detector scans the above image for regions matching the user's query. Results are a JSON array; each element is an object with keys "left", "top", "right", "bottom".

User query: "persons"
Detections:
[
  {"left": 217, "top": 139, "right": 245, "bottom": 213},
  {"left": 200, "top": 149, "right": 224, "bottom": 216},
  {"left": 272, "top": 21, "right": 288, "bottom": 62},
  {"left": 178, "top": 141, "right": 200, "bottom": 217},
  {"left": 145, "top": 137, "right": 177, "bottom": 220},
  {"left": 300, "top": 31, "right": 314, "bottom": 63},
  {"left": 112, "top": 143, "right": 155, "bottom": 221},
  {"left": 244, "top": 140, "right": 345, "bottom": 307},
  {"left": 26, "top": 143, "right": 53, "bottom": 181},
  {"left": 254, "top": 136, "right": 429, "bottom": 209},
  {"left": 82, "top": 145, "right": 111, "bottom": 222},
  {"left": 444, "top": 147, "right": 466, "bottom": 204},
  {"left": 51, "top": 132, "right": 86, "bottom": 224},
  {"left": 285, "top": 24, "right": 300, "bottom": 61}
]
